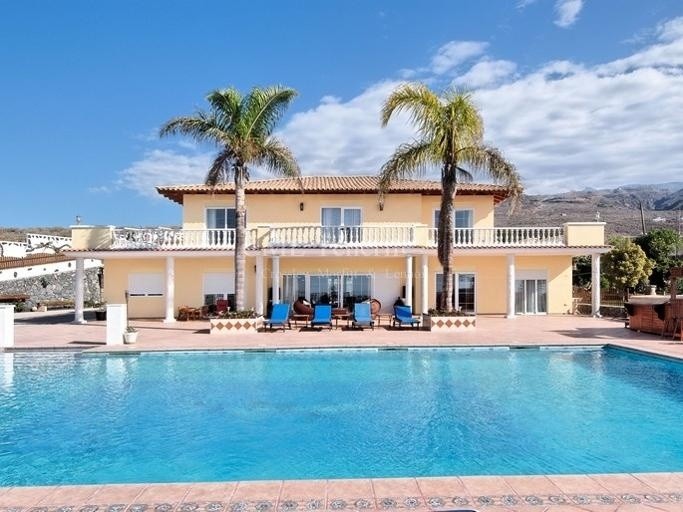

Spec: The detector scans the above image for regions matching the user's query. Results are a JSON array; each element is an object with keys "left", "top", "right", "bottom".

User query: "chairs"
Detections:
[
  {"left": 263, "top": 303, "right": 421, "bottom": 333},
  {"left": 176, "top": 299, "right": 229, "bottom": 321}
]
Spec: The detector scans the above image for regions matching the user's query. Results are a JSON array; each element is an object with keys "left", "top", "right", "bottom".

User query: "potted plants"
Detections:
[{"left": 123, "top": 326, "right": 138, "bottom": 344}]
